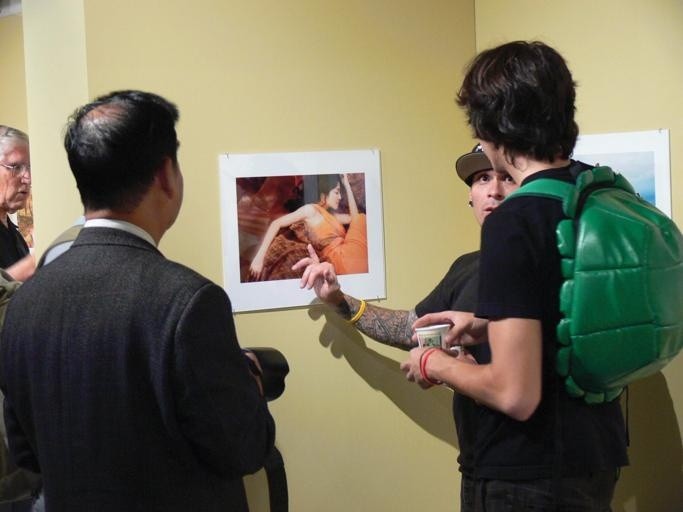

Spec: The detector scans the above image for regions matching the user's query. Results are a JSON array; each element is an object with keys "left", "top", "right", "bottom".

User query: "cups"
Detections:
[{"left": 413, "top": 322, "right": 452, "bottom": 353}]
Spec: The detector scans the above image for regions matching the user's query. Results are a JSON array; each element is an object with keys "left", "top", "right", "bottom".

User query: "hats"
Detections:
[{"left": 455, "top": 141, "right": 494, "bottom": 187}]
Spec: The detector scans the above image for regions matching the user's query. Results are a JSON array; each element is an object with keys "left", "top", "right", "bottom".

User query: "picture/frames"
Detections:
[
  {"left": 572, "top": 128, "right": 674, "bottom": 223},
  {"left": 218, "top": 148, "right": 385, "bottom": 316}
]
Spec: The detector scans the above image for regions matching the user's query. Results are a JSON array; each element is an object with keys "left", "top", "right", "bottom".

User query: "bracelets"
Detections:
[
  {"left": 422, "top": 347, "right": 446, "bottom": 387},
  {"left": 417, "top": 345, "right": 432, "bottom": 375},
  {"left": 349, "top": 298, "right": 367, "bottom": 326}
]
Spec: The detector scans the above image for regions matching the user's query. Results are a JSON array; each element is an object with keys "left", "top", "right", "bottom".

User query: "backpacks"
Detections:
[
  {"left": 0, "top": 224, "right": 85, "bottom": 335},
  {"left": 504, "top": 166, "right": 683, "bottom": 404}
]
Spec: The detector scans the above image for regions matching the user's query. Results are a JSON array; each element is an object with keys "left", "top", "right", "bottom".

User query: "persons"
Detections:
[
  {"left": 0, "top": 124, "right": 41, "bottom": 512},
  {"left": 0, "top": 92, "right": 276, "bottom": 512},
  {"left": 291, "top": 144, "right": 519, "bottom": 512},
  {"left": 397, "top": 38, "right": 630, "bottom": 512},
  {"left": 247, "top": 173, "right": 367, "bottom": 285}
]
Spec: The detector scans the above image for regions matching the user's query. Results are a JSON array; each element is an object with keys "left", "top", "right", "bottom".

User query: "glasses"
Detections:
[{"left": 0, "top": 162, "right": 30, "bottom": 178}]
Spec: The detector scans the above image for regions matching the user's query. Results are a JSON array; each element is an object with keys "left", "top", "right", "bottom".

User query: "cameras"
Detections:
[{"left": 241, "top": 347, "right": 289, "bottom": 402}]
